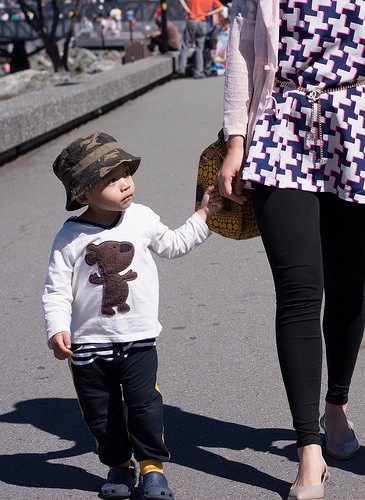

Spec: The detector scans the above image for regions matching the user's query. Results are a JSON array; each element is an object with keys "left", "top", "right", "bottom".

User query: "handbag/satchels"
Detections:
[{"left": 195, "top": 128, "right": 262, "bottom": 240}]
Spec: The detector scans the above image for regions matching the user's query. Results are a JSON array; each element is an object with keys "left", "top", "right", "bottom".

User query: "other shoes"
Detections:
[
  {"left": 288, "top": 464, "right": 331, "bottom": 499},
  {"left": 320, "top": 414, "right": 359, "bottom": 459}
]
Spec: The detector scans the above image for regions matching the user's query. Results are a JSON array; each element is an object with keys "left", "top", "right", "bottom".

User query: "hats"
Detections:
[{"left": 53, "top": 132, "right": 141, "bottom": 211}]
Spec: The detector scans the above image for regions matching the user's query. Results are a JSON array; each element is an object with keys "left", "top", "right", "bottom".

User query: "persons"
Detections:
[
  {"left": 0, "top": 7, "right": 230, "bottom": 79},
  {"left": 172, "top": 0, "right": 224, "bottom": 79},
  {"left": 218, "top": 0, "right": 365, "bottom": 500},
  {"left": 43, "top": 132, "right": 226, "bottom": 500}
]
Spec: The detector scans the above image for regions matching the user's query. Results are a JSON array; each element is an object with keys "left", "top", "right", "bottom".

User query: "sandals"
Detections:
[
  {"left": 138, "top": 471, "right": 174, "bottom": 500},
  {"left": 99, "top": 460, "right": 138, "bottom": 499}
]
